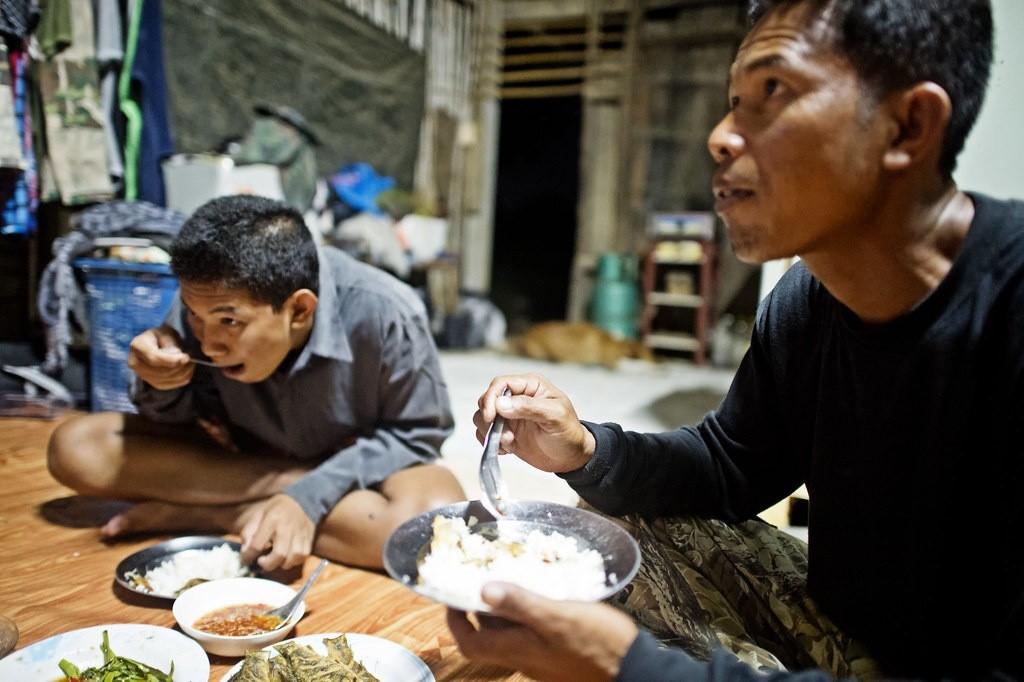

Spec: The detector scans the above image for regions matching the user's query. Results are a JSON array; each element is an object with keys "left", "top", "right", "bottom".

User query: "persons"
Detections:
[
  {"left": 48, "top": 193, "right": 468, "bottom": 573},
  {"left": 450, "top": 1, "right": 1024, "bottom": 682}
]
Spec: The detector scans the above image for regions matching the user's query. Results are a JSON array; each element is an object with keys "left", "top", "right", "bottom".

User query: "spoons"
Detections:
[
  {"left": 268, "top": 556, "right": 329, "bottom": 629},
  {"left": 473, "top": 382, "right": 514, "bottom": 518},
  {"left": 186, "top": 356, "right": 240, "bottom": 368}
]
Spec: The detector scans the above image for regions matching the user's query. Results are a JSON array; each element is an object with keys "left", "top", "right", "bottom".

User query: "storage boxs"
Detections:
[{"left": 76, "top": 255, "right": 187, "bottom": 417}]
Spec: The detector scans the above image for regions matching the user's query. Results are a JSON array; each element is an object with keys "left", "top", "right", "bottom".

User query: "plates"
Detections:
[
  {"left": 0, "top": 622, "right": 212, "bottom": 682},
  {"left": 219, "top": 631, "right": 438, "bottom": 682},
  {"left": 114, "top": 533, "right": 269, "bottom": 601},
  {"left": 0, "top": 611, "right": 20, "bottom": 660}
]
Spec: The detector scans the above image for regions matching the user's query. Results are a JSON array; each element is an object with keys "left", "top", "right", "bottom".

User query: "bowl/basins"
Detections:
[
  {"left": 382, "top": 500, "right": 643, "bottom": 617},
  {"left": 173, "top": 576, "right": 305, "bottom": 656}
]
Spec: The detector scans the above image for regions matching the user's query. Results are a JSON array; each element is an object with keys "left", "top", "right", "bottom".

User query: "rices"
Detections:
[
  {"left": 414, "top": 512, "right": 608, "bottom": 608},
  {"left": 120, "top": 542, "right": 246, "bottom": 594}
]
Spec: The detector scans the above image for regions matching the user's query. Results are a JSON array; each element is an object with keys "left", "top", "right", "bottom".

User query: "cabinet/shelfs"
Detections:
[{"left": 638, "top": 214, "right": 717, "bottom": 362}]
